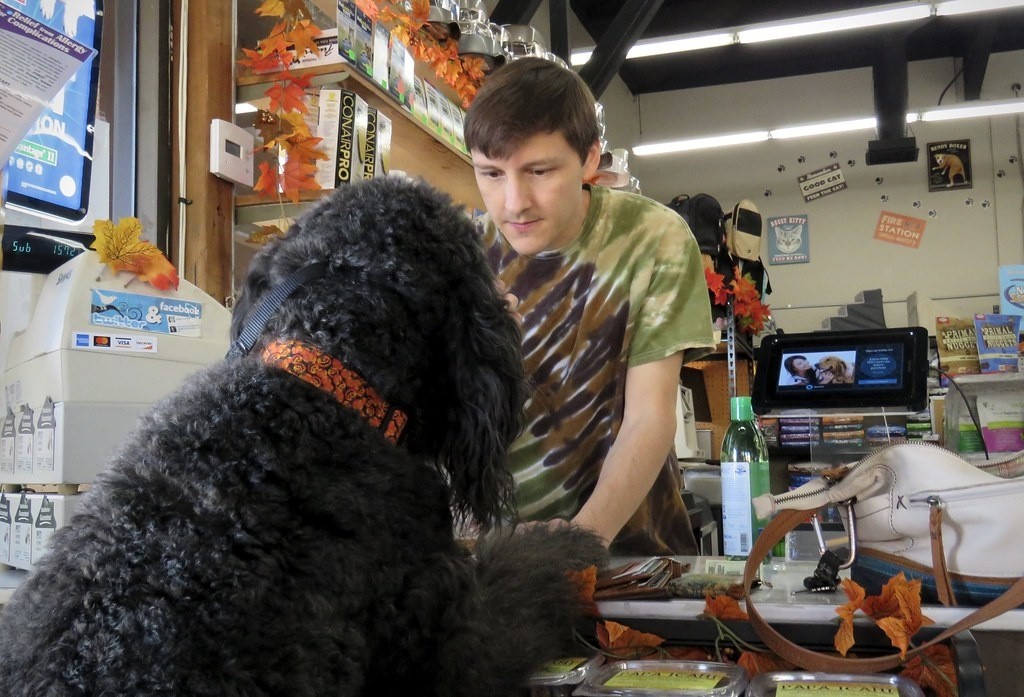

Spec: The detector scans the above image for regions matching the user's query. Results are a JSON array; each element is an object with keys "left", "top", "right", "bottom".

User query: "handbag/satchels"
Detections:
[
  {"left": 743, "top": 441, "right": 1022, "bottom": 669},
  {"left": 724, "top": 198, "right": 763, "bottom": 261},
  {"left": 666, "top": 192, "right": 724, "bottom": 252}
]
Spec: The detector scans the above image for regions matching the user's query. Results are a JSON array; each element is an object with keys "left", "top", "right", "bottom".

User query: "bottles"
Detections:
[{"left": 720, "top": 396, "right": 773, "bottom": 564}]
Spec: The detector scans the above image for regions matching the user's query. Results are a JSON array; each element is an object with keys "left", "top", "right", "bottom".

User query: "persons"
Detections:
[
  {"left": 450, "top": 56, "right": 713, "bottom": 558},
  {"left": 784, "top": 356, "right": 835, "bottom": 386}
]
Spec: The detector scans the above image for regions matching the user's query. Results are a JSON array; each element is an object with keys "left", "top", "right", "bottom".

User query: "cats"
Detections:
[{"left": 774, "top": 224, "right": 803, "bottom": 253}]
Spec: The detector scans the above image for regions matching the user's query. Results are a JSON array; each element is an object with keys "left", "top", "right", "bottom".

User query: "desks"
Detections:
[{"left": 584, "top": 552, "right": 1023, "bottom": 697}]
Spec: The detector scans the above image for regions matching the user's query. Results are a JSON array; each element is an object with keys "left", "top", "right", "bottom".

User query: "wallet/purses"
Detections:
[{"left": 592, "top": 555, "right": 682, "bottom": 602}]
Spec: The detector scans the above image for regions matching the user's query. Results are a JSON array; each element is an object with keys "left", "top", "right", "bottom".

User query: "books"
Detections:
[
  {"left": 769, "top": 416, "right": 931, "bottom": 521},
  {"left": 934, "top": 265, "right": 1024, "bottom": 377}
]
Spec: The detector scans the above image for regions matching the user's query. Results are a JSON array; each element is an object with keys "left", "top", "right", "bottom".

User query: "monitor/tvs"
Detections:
[
  {"left": 751, "top": 327, "right": 929, "bottom": 416},
  {"left": 0, "top": 0, "right": 105, "bottom": 223}
]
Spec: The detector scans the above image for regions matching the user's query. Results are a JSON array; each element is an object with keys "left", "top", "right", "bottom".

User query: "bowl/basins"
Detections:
[
  {"left": 518, "top": 653, "right": 604, "bottom": 697},
  {"left": 745, "top": 671, "right": 925, "bottom": 697},
  {"left": 572, "top": 659, "right": 749, "bottom": 697}
]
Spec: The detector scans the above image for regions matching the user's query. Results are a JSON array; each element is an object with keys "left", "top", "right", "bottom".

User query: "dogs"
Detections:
[
  {"left": 932, "top": 153, "right": 968, "bottom": 187},
  {"left": 0, "top": 177, "right": 612, "bottom": 697},
  {"left": 814, "top": 355, "right": 854, "bottom": 383}
]
